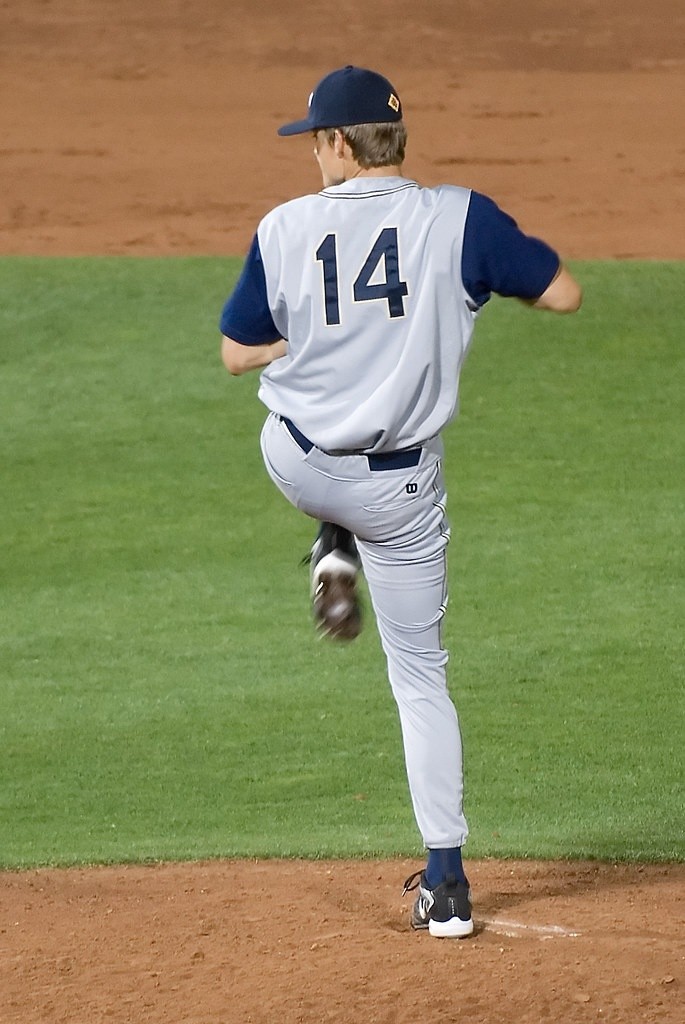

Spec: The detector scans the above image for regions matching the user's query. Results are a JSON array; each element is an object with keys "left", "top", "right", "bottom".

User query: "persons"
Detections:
[{"left": 218, "top": 65, "right": 581, "bottom": 940}]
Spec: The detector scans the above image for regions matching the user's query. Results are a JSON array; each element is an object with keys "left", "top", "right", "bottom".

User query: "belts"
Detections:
[{"left": 282, "top": 417, "right": 422, "bottom": 471}]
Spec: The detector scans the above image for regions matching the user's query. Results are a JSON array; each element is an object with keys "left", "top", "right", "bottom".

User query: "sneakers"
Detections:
[
  {"left": 402, "top": 869, "right": 474, "bottom": 938},
  {"left": 298, "top": 522, "right": 363, "bottom": 647}
]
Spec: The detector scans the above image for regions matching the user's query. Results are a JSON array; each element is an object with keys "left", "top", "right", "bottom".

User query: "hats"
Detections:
[{"left": 278, "top": 66, "right": 402, "bottom": 136}]
